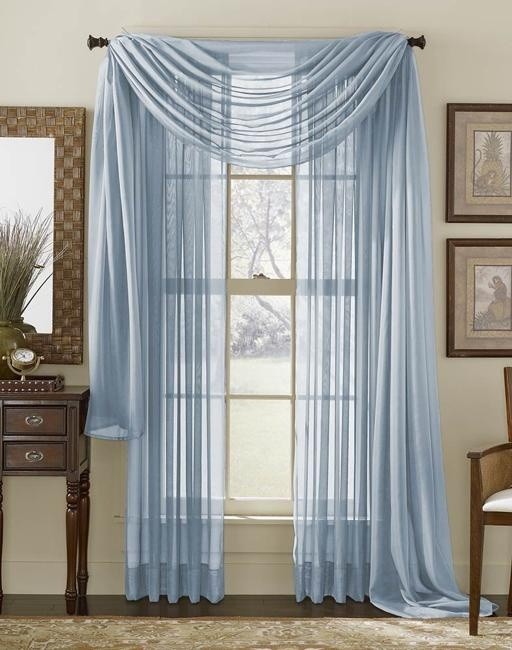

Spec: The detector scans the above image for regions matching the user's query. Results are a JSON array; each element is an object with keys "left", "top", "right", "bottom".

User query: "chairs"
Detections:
[{"left": 465, "top": 367, "right": 511, "bottom": 636}]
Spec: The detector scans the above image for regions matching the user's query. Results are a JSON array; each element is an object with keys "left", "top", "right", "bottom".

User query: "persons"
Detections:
[{"left": 488, "top": 275, "right": 508, "bottom": 301}]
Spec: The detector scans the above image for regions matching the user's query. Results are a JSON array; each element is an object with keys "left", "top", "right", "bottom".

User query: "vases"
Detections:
[
  {"left": 15, "top": 317, "right": 37, "bottom": 332},
  {"left": 1, "top": 320, "right": 28, "bottom": 378}
]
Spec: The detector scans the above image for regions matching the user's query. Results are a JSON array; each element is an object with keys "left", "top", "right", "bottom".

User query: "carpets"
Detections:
[{"left": 0, "top": 615, "right": 511, "bottom": 649}]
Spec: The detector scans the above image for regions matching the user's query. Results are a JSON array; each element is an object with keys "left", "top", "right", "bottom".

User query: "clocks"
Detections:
[{"left": 2, "top": 348, "right": 45, "bottom": 380}]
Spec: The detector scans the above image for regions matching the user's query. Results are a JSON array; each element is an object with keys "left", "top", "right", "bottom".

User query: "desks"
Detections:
[{"left": 1, "top": 386, "right": 92, "bottom": 616}]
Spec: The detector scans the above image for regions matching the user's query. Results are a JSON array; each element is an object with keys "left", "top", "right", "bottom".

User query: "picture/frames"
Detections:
[{"left": 445, "top": 103, "right": 511, "bottom": 358}]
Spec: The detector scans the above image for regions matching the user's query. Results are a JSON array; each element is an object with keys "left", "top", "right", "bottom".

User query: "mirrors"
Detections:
[{"left": 0, "top": 105, "right": 86, "bottom": 365}]
